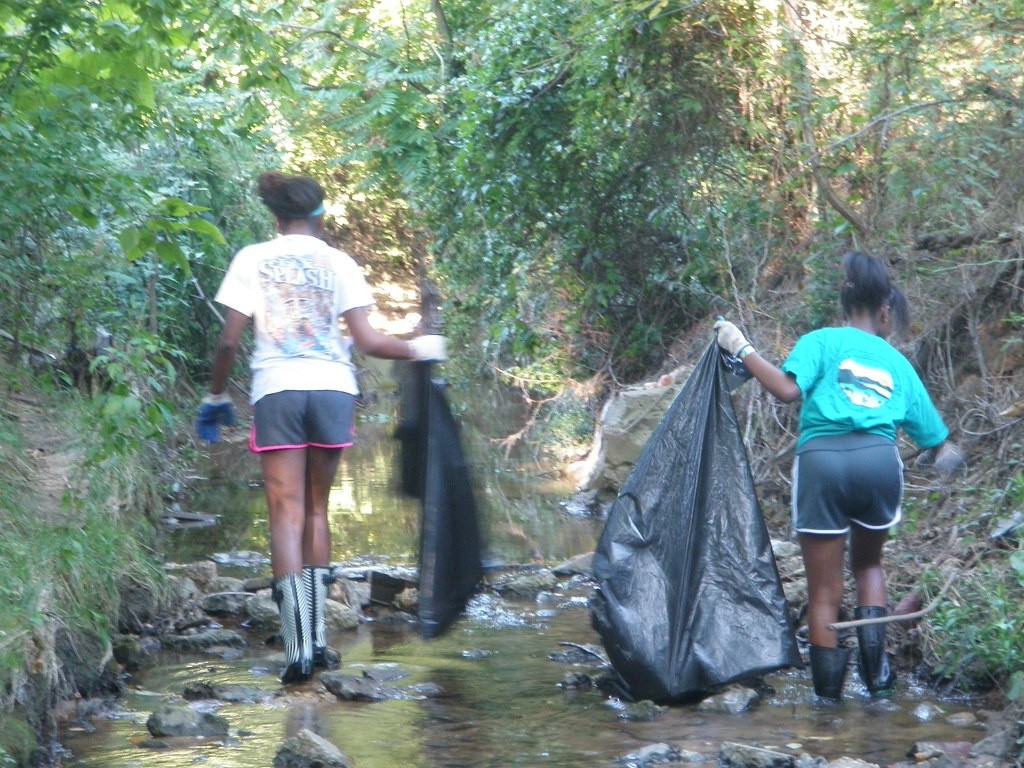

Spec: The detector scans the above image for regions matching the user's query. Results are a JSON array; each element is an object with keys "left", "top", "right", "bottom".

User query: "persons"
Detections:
[
  {"left": 713, "top": 252, "right": 948, "bottom": 703},
  {"left": 192, "top": 172, "right": 449, "bottom": 685}
]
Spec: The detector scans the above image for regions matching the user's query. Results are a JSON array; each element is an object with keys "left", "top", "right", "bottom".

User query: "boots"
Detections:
[
  {"left": 809, "top": 644, "right": 850, "bottom": 698},
  {"left": 302, "top": 566, "right": 340, "bottom": 667},
  {"left": 854, "top": 606, "right": 891, "bottom": 692},
  {"left": 271, "top": 573, "right": 313, "bottom": 684}
]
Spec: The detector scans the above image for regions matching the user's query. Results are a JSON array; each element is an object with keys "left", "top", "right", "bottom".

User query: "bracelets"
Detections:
[
  {"left": 736, "top": 345, "right": 756, "bottom": 361},
  {"left": 406, "top": 340, "right": 415, "bottom": 358},
  {"left": 207, "top": 391, "right": 226, "bottom": 400}
]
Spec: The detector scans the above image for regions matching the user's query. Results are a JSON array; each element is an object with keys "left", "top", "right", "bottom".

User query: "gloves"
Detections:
[
  {"left": 405, "top": 336, "right": 448, "bottom": 364},
  {"left": 195, "top": 392, "right": 235, "bottom": 444},
  {"left": 713, "top": 320, "right": 750, "bottom": 358}
]
